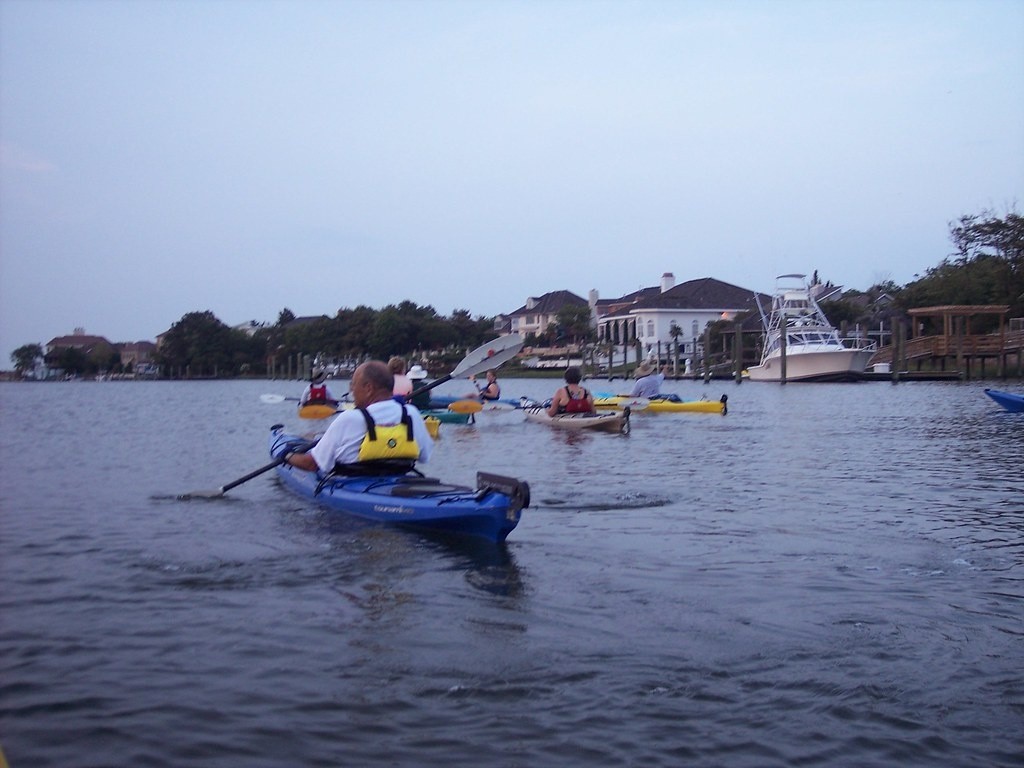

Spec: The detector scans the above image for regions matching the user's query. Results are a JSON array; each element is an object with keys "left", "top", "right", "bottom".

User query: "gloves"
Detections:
[{"left": 281, "top": 446, "right": 294, "bottom": 463}]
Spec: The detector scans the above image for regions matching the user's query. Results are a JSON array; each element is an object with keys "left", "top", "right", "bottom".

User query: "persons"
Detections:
[
  {"left": 294, "top": 358, "right": 706, "bottom": 415},
  {"left": 276, "top": 361, "right": 435, "bottom": 477}
]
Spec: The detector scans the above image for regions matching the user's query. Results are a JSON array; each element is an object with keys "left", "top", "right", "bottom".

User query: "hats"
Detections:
[
  {"left": 406, "top": 365, "right": 428, "bottom": 379},
  {"left": 637, "top": 361, "right": 655, "bottom": 376},
  {"left": 311, "top": 368, "right": 329, "bottom": 384}
]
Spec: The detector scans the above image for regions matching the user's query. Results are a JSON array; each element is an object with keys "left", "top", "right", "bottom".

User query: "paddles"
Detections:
[
  {"left": 299, "top": 400, "right": 483, "bottom": 419},
  {"left": 482, "top": 397, "right": 649, "bottom": 417},
  {"left": 262, "top": 392, "right": 355, "bottom": 404},
  {"left": 476, "top": 359, "right": 507, "bottom": 400},
  {"left": 594, "top": 391, "right": 694, "bottom": 403},
  {"left": 185, "top": 334, "right": 528, "bottom": 499}
]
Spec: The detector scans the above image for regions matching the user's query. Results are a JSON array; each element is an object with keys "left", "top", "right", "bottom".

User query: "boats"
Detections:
[
  {"left": 982, "top": 386, "right": 1024, "bottom": 415},
  {"left": 747, "top": 272, "right": 879, "bottom": 383},
  {"left": 269, "top": 425, "right": 530, "bottom": 544},
  {"left": 297, "top": 390, "right": 730, "bottom": 433}
]
